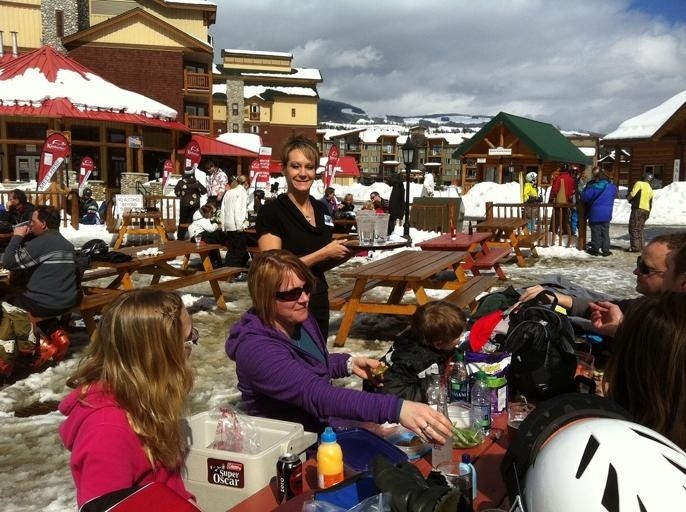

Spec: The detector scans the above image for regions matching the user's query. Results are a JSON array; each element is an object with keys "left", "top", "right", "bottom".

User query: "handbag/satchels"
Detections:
[{"left": 1, "top": 301, "right": 42, "bottom": 381}]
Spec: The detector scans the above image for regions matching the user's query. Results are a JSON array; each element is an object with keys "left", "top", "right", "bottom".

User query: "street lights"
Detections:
[{"left": 401, "top": 133, "right": 415, "bottom": 238}]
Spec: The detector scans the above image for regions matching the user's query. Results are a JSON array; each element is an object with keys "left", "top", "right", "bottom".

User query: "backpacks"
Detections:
[
  {"left": 468, "top": 285, "right": 521, "bottom": 324},
  {"left": 180, "top": 179, "right": 200, "bottom": 208}
]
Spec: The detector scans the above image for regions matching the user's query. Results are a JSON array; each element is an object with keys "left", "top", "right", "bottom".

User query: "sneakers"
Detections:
[
  {"left": 52, "top": 331, "right": 70, "bottom": 362},
  {"left": 30, "top": 337, "right": 58, "bottom": 370}
]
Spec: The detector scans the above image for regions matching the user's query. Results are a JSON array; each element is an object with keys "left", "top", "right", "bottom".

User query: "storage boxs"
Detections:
[{"left": 177, "top": 410, "right": 318, "bottom": 511}]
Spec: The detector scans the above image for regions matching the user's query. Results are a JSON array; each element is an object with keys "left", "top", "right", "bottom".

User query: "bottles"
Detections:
[
  {"left": 460, "top": 453, "right": 477, "bottom": 501},
  {"left": 431, "top": 393, "right": 453, "bottom": 468},
  {"left": 426, "top": 374, "right": 442, "bottom": 404},
  {"left": 444, "top": 361, "right": 454, "bottom": 389},
  {"left": 316, "top": 426, "right": 345, "bottom": 492},
  {"left": 450, "top": 354, "right": 468, "bottom": 403},
  {"left": 470, "top": 370, "right": 492, "bottom": 437},
  {"left": 276, "top": 452, "right": 303, "bottom": 505}
]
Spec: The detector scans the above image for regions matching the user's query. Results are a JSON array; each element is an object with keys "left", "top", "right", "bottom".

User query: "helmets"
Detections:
[
  {"left": 570, "top": 164, "right": 582, "bottom": 177},
  {"left": 641, "top": 172, "right": 654, "bottom": 185},
  {"left": 507, "top": 407, "right": 686, "bottom": 511},
  {"left": 83, "top": 188, "right": 93, "bottom": 198},
  {"left": 557, "top": 162, "right": 570, "bottom": 174},
  {"left": 526, "top": 173, "right": 537, "bottom": 185},
  {"left": 592, "top": 165, "right": 603, "bottom": 179},
  {"left": 81, "top": 239, "right": 109, "bottom": 262}
]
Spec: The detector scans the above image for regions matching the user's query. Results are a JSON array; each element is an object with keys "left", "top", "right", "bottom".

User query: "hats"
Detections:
[{"left": 184, "top": 167, "right": 195, "bottom": 179}]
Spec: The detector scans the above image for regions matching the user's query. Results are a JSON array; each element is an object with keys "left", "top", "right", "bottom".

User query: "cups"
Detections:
[
  {"left": 355, "top": 210, "right": 375, "bottom": 242},
  {"left": 194, "top": 230, "right": 202, "bottom": 248},
  {"left": 11, "top": 221, "right": 30, "bottom": 233},
  {"left": 437, "top": 462, "right": 471, "bottom": 503},
  {"left": 507, "top": 402, "right": 535, "bottom": 444},
  {"left": 374, "top": 213, "right": 390, "bottom": 242}
]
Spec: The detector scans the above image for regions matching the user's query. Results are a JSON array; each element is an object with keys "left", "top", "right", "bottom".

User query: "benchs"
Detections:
[
  {"left": 30, "top": 293, "right": 117, "bottom": 322},
  {"left": 440, "top": 277, "right": 497, "bottom": 309},
  {"left": 82, "top": 268, "right": 117, "bottom": 282},
  {"left": 473, "top": 247, "right": 512, "bottom": 270},
  {"left": 327, "top": 279, "right": 383, "bottom": 312},
  {"left": 222, "top": 245, "right": 259, "bottom": 254},
  {"left": 163, "top": 219, "right": 177, "bottom": 233},
  {"left": 106, "top": 219, "right": 121, "bottom": 233},
  {"left": 517, "top": 233, "right": 545, "bottom": 248},
  {"left": 145, "top": 267, "right": 246, "bottom": 292},
  {"left": 332, "top": 233, "right": 351, "bottom": 240}
]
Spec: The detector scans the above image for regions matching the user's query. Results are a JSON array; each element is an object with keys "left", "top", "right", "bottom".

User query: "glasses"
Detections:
[
  {"left": 371, "top": 194, "right": 377, "bottom": 200},
  {"left": 175, "top": 328, "right": 200, "bottom": 350},
  {"left": 276, "top": 282, "right": 315, "bottom": 302},
  {"left": 637, "top": 255, "right": 678, "bottom": 276}
]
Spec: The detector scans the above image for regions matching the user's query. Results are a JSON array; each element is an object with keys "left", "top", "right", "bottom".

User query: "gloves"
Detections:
[{"left": 369, "top": 449, "right": 473, "bottom": 512}]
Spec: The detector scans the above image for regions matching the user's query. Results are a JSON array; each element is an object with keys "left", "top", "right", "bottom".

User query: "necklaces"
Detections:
[
  {"left": 287, "top": 191, "right": 312, "bottom": 223},
  {"left": 275, "top": 320, "right": 301, "bottom": 349}
]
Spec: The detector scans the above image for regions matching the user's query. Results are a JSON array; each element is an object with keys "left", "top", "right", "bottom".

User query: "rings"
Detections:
[{"left": 423, "top": 424, "right": 430, "bottom": 431}]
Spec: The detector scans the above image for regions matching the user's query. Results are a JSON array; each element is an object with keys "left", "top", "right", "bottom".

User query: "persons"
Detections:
[
  {"left": 207, "top": 161, "right": 228, "bottom": 201},
  {"left": 225, "top": 250, "right": 453, "bottom": 457},
  {"left": 188, "top": 206, "right": 233, "bottom": 247},
  {"left": 59, "top": 287, "right": 205, "bottom": 512},
  {"left": 206, "top": 200, "right": 222, "bottom": 266},
  {"left": 507, "top": 387, "right": 685, "bottom": 507},
  {"left": 339, "top": 194, "right": 354, "bottom": 212},
  {"left": 320, "top": 187, "right": 334, "bottom": 216},
  {"left": 1, "top": 189, "right": 36, "bottom": 223},
  {"left": 524, "top": 172, "right": 538, "bottom": 235},
  {"left": 174, "top": 166, "right": 207, "bottom": 241},
  {"left": 78, "top": 188, "right": 98, "bottom": 219},
  {"left": 364, "top": 301, "right": 464, "bottom": 405},
  {"left": 98, "top": 187, "right": 110, "bottom": 223},
  {"left": 585, "top": 167, "right": 602, "bottom": 245},
  {"left": 581, "top": 168, "right": 617, "bottom": 257},
  {"left": 257, "top": 130, "right": 352, "bottom": 344},
  {"left": 572, "top": 170, "right": 586, "bottom": 238},
  {"left": 548, "top": 165, "right": 574, "bottom": 234},
  {"left": 0, "top": 205, "right": 78, "bottom": 371},
  {"left": 519, "top": 232, "right": 686, "bottom": 320},
  {"left": 422, "top": 172, "right": 436, "bottom": 197},
  {"left": 587, "top": 300, "right": 686, "bottom": 452},
  {"left": 625, "top": 171, "right": 654, "bottom": 253},
  {"left": 220, "top": 176, "right": 250, "bottom": 264},
  {"left": 361, "top": 200, "right": 374, "bottom": 210},
  {"left": 248, "top": 190, "right": 266, "bottom": 214},
  {"left": 81, "top": 206, "right": 101, "bottom": 225},
  {"left": 370, "top": 192, "right": 390, "bottom": 211}
]
